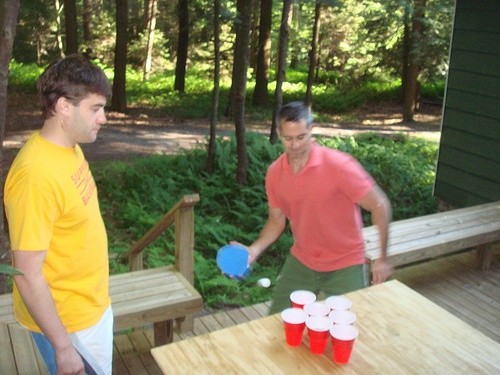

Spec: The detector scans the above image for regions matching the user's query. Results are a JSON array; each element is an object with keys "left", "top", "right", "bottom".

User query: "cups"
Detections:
[
  {"left": 289, "top": 290, "right": 317, "bottom": 309},
  {"left": 326, "top": 296, "right": 359, "bottom": 364},
  {"left": 281, "top": 307, "right": 309, "bottom": 346},
  {"left": 303, "top": 301, "right": 333, "bottom": 354}
]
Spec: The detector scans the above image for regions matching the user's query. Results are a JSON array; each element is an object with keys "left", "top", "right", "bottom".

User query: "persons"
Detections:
[
  {"left": 222, "top": 102, "right": 392, "bottom": 316},
  {"left": 4, "top": 56, "right": 114, "bottom": 375}
]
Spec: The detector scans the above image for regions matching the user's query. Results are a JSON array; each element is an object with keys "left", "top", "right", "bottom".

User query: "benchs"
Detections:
[
  {"left": 364, "top": 199, "right": 500, "bottom": 285},
  {"left": 0, "top": 264, "right": 204, "bottom": 375}
]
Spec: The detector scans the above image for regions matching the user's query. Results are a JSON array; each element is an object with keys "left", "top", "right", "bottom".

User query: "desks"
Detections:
[{"left": 150, "top": 277, "right": 500, "bottom": 374}]
